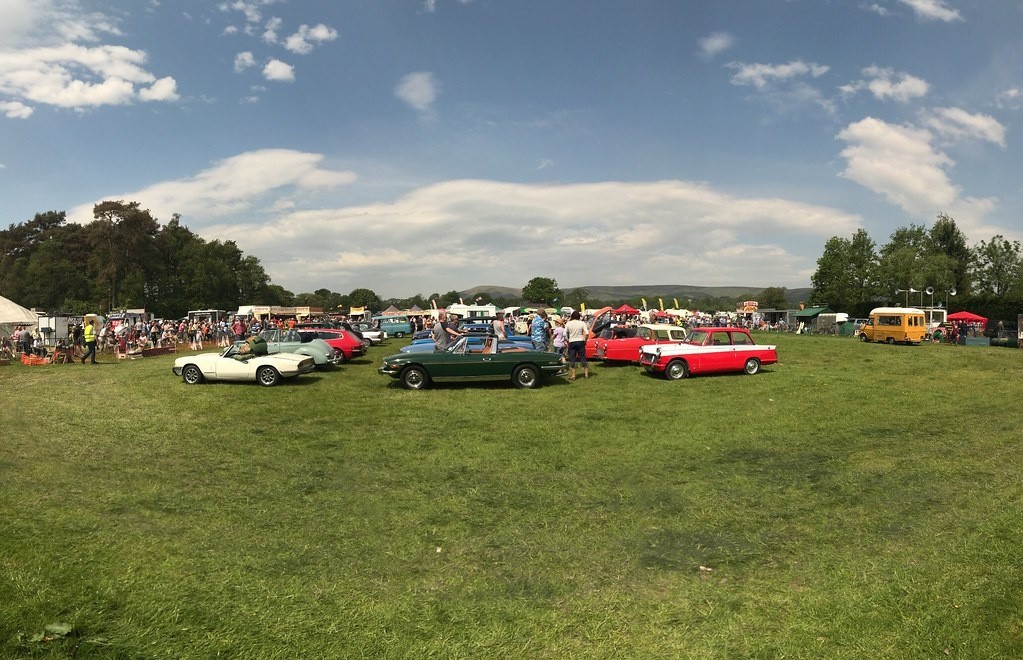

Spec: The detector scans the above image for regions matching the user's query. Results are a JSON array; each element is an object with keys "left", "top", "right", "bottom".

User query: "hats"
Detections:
[
  {"left": 553, "top": 318, "right": 563, "bottom": 325},
  {"left": 238, "top": 316, "right": 244, "bottom": 321}
]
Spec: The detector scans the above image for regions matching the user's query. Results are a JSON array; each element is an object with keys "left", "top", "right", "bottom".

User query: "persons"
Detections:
[
  {"left": 565, "top": 311, "right": 589, "bottom": 380},
  {"left": 68, "top": 314, "right": 362, "bottom": 356},
  {"left": 997, "top": 321, "right": 1003, "bottom": 338},
  {"left": 1, "top": 325, "right": 41, "bottom": 358},
  {"left": 598, "top": 314, "right": 785, "bottom": 333},
  {"left": 531, "top": 308, "right": 547, "bottom": 351},
  {"left": 410, "top": 312, "right": 462, "bottom": 348},
  {"left": 940, "top": 320, "right": 975, "bottom": 346},
  {"left": 527, "top": 314, "right": 571, "bottom": 363},
  {"left": 81, "top": 320, "right": 100, "bottom": 364},
  {"left": 490, "top": 312, "right": 511, "bottom": 341}
]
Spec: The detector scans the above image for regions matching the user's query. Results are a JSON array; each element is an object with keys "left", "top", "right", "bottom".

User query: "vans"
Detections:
[
  {"left": 859, "top": 307, "right": 927, "bottom": 345},
  {"left": 371, "top": 315, "right": 562, "bottom": 339}
]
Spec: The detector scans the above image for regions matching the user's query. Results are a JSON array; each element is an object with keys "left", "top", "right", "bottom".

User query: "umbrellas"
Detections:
[{"left": 521, "top": 306, "right": 575, "bottom": 315}]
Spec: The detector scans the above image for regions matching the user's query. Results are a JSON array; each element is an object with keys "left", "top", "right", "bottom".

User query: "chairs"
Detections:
[
  {"left": 712, "top": 339, "right": 721, "bottom": 345},
  {"left": 482, "top": 334, "right": 498, "bottom": 354},
  {"left": 116, "top": 347, "right": 126, "bottom": 363}
]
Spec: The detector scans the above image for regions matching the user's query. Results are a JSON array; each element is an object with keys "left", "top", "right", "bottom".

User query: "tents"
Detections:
[
  {"left": 615, "top": 304, "right": 640, "bottom": 314},
  {"left": 947, "top": 311, "right": 987, "bottom": 327},
  {"left": 0, "top": 296, "right": 41, "bottom": 341},
  {"left": 383, "top": 304, "right": 431, "bottom": 317}
]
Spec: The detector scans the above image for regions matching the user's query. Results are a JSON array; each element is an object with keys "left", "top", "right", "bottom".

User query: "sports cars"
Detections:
[
  {"left": 223, "top": 329, "right": 343, "bottom": 370},
  {"left": 172, "top": 341, "right": 316, "bottom": 386},
  {"left": 593, "top": 325, "right": 690, "bottom": 362},
  {"left": 376, "top": 334, "right": 569, "bottom": 390},
  {"left": 397, "top": 332, "right": 534, "bottom": 352}
]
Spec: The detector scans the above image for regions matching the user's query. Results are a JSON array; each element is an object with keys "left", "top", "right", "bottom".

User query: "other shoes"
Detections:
[
  {"left": 92, "top": 361, "right": 99, "bottom": 364},
  {"left": 569, "top": 377, "right": 576, "bottom": 381},
  {"left": 81, "top": 358, "right": 85, "bottom": 365}
]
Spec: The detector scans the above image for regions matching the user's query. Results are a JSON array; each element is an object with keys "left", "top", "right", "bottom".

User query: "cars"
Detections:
[
  {"left": 639, "top": 327, "right": 779, "bottom": 380},
  {"left": 566, "top": 320, "right": 627, "bottom": 361},
  {"left": 291, "top": 330, "right": 369, "bottom": 365},
  {"left": 292, "top": 320, "right": 390, "bottom": 348}
]
